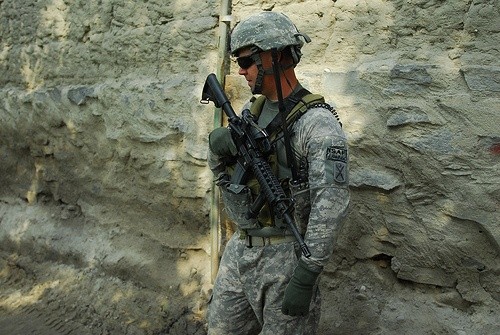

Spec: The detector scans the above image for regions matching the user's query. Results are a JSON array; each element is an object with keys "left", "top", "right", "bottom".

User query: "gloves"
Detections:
[
  {"left": 281, "top": 259, "right": 319, "bottom": 316},
  {"left": 208, "top": 127, "right": 239, "bottom": 158}
]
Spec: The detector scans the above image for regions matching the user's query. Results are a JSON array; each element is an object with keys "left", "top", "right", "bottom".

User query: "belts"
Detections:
[{"left": 237, "top": 229, "right": 297, "bottom": 248}]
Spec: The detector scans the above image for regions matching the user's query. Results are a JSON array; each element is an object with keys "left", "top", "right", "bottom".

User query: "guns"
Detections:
[{"left": 200, "top": 73, "right": 312, "bottom": 258}]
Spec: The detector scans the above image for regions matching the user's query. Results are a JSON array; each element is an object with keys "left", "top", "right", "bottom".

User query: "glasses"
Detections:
[{"left": 237, "top": 56, "right": 255, "bottom": 68}]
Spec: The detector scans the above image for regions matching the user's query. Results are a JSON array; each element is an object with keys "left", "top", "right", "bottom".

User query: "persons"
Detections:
[{"left": 205, "top": 12, "right": 352, "bottom": 335}]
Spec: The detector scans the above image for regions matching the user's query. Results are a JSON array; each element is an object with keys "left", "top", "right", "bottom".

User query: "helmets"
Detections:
[{"left": 231, "top": 11, "right": 310, "bottom": 51}]
